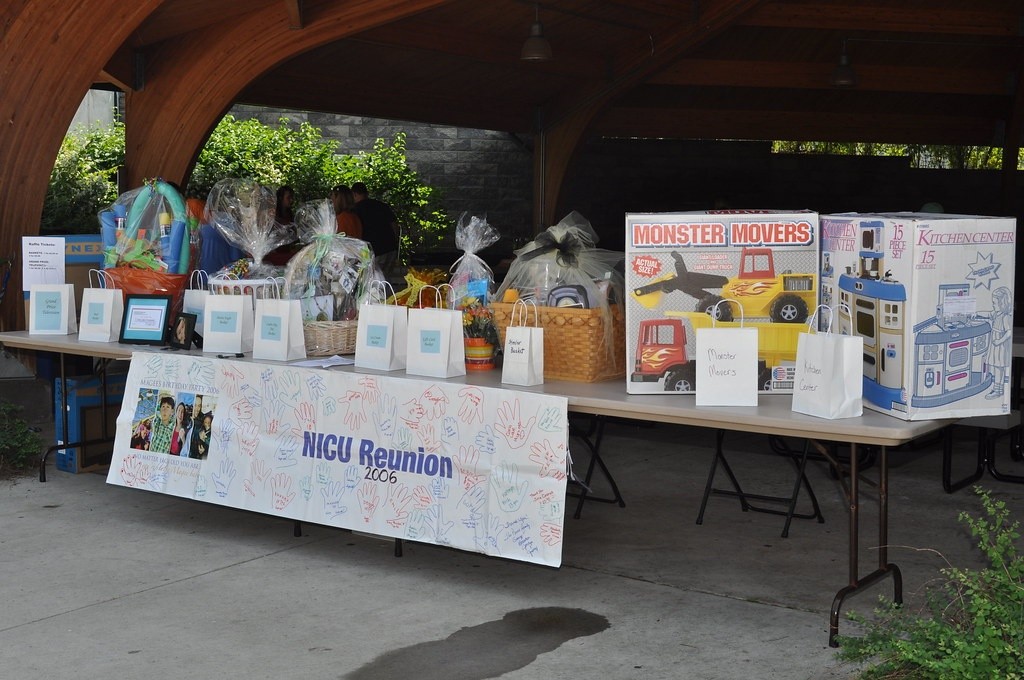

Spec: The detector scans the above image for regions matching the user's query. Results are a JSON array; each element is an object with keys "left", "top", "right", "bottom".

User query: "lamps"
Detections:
[{"left": 520, "top": 0, "right": 552, "bottom": 62}]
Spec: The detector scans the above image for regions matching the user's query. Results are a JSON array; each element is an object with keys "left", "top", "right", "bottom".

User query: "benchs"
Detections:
[{"left": 768, "top": 410, "right": 1023, "bottom": 493}]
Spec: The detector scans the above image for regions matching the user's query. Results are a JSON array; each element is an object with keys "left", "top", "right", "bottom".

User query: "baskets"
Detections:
[
  {"left": 304, "top": 319, "right": 359, "bottom": 356},
  {"left": 490, "top": 300, "right": 627, "bottom": 382}
]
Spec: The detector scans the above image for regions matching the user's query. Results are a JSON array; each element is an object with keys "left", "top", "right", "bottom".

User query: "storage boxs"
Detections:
[
  {"left": 625, "top": 210, "right": 819, "bottom": 395},
  {"left": 55, "top": 371, "right": 128, "bottom": 474},
  {"left": 23, "top": 235, "right": 105, "bottom": 331},
  {"left": 818, "top": 212, "right": 1016, "bottom": 421}
]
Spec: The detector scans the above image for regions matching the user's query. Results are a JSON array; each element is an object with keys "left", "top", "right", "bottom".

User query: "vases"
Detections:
[{"left": 464, "top": 337, "right": 496, "bottom": 371}]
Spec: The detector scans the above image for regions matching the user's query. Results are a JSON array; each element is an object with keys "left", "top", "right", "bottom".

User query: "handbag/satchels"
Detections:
[
  {"left": 354, "top": 280, "right": 407, "bottom": 371},
  {"left": 406, "top": 284, "right": 467, "bottom": 378},
  {"left": 501, "top": 299, "right": 544, "bottom": 385},
  {"left": 104, "top": 259, "right": 191, "bottom": 327},
  {"left": 251, "top": 277, "right": 307, "bottom": 361},
  {"left": 696, "top": 299, "right": 759, "bottom": 407},
  {"left": 78, "top": 269, "right": 124, "bottom": 341},
  {"left": 29, "top": 264, "right": 78, "bottom": 335},
  {"left": 182, "top": 269, "right": 211, "bottom": 345},
  {"left": 791, "top": 305, "right": 863, "bottom": 417},
  {"left": 203, "top": 274, "right": 255, "bottom": 353}
]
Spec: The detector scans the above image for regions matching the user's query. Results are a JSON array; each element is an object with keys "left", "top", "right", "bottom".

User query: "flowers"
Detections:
[{"left": 455, "top": 296, "right": 501, "bottom": 363}]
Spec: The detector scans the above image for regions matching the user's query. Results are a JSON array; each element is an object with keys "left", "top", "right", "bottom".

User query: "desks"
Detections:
[{"left": 1, "top": 332, "right": 963, "bottom": 648}]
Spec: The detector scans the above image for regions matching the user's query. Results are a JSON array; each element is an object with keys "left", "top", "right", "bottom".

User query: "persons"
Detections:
[
  {"left": 175, "top": 317, "right": 188, "bottom": 342},
  {"left": 329, "top": 184, "right": 362, "bottom": 238},
  {"left": 199, "top": 213, "right": 248, "bottom": 285},
  {"left": 352, "top": 182, "right": 399, "bottom": 274},
  {"left": 167, "top": 181, "right": 210, "bottom": 226},
  {"left": 274, "top": 185, "right": 296, "bottom": 253},
  {"left": 131, "top": 394, "right": 213, "bottom": 460}
]
